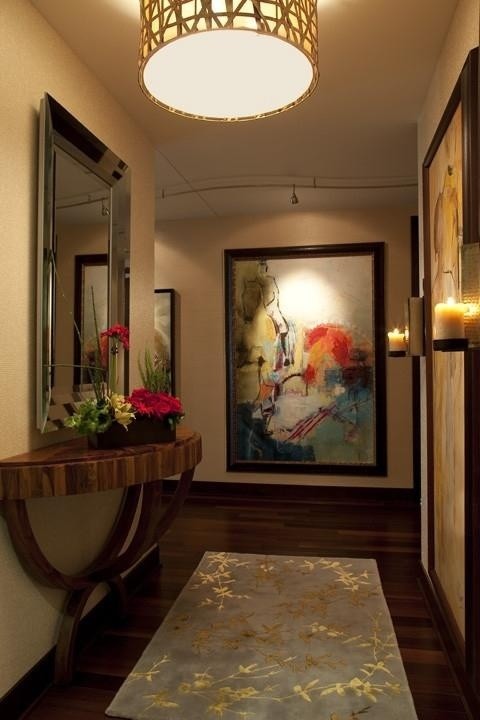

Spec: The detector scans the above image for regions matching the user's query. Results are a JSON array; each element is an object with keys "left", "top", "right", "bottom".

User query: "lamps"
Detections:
[
  {"left": 101, "top": 199, "right": 110, "bottom": 216},
  {"left": 134, "top": 0, "right": 319, "bottom": 123},
  {"left": 288, "top": 184, "right": 299, "bottom": 204}
]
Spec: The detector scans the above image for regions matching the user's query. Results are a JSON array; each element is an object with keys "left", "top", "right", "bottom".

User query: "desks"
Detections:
[{"left": 1, "top": 432, "right": 202, "bottom": 691}]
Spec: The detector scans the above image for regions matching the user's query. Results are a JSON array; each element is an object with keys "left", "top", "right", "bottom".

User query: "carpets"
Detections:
[{"left": 103, "top": 550, "right": 418, "bottom": 720}]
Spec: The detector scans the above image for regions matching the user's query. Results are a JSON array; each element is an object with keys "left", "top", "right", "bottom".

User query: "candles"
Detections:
[
  {"left": 387, "top": 331, "right": 406, "bottom": 353},
  {"left": 432, "top": 300, "right": 465, "bottom": 339}
]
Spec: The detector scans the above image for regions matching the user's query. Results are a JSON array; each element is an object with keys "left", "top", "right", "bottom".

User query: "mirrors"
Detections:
[{"left": 36, "top": 90, "right": 132, "bottom": 434}]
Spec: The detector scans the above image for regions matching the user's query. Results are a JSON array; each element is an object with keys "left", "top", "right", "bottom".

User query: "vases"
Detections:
[{"left": 87, "top": 415, "right": 176, "bottom": 450}]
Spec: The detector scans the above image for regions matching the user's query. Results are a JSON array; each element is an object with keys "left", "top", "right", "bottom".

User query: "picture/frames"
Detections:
[
  {"left": 421, "top": 46, "right": 479, "bottom": 694},
  {"left": 73, "top": 253, "right": 109, "bottom": 392},
  {"left": 221, "top": 238, "right": 389, "bottom": 478}
]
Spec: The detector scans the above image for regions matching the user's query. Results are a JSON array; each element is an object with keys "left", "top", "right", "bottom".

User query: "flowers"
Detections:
[{"left": 44, "top": 249, "right": 188, "bottom": 433}]
